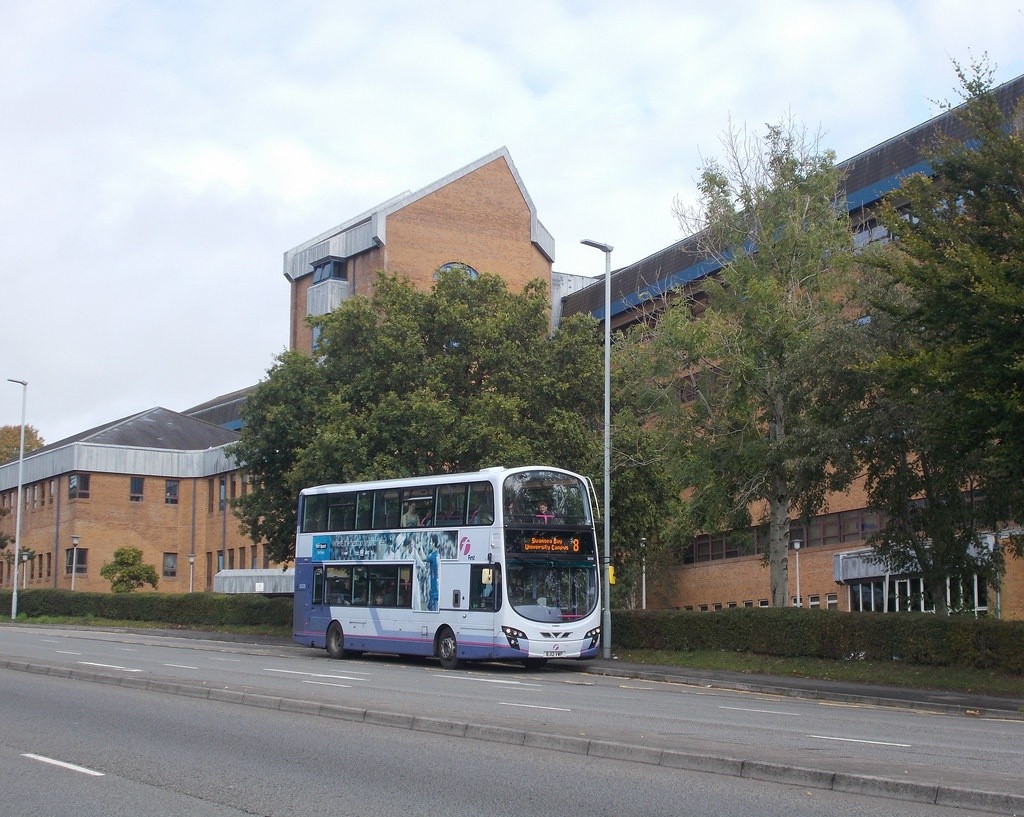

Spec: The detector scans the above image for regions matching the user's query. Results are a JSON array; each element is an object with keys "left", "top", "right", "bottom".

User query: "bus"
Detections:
[{"left": 292, "top": 464, "right": 605, "bottom": 672}]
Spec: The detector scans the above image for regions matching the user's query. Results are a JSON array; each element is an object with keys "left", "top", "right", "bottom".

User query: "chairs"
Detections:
[{"left": 302, "top": 499, "right": 505, "bottom": 611}]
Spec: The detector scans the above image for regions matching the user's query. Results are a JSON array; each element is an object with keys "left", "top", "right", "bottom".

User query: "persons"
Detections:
[
  {"left": 401, "top": 497, "right": 565, "bottom": 528},
  {"left": 334, "top": 575, "right": 412, "bottom": 607}
]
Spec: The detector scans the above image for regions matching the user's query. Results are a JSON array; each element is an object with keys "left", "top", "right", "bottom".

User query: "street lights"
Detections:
[
  {"left": 187, "top": 553, "right": 198, "bottom": 593},
  {"left": 639, "top": 538, "right": 649, "bottom": 609},
  {"left": 71, "top": 534, "right": 82, "bottom": 592},
  {"left": 20, "top": 549, "right": 30, "bottom": 589},
  {"left": 6, "top": 378, "right": 29, "bottom": 620},
  {"left": 579, "top": 238, "right": 624, "bottom": 659},
  {"left": 791, "top": 538, "right": 803, "bottom": 606}
]
